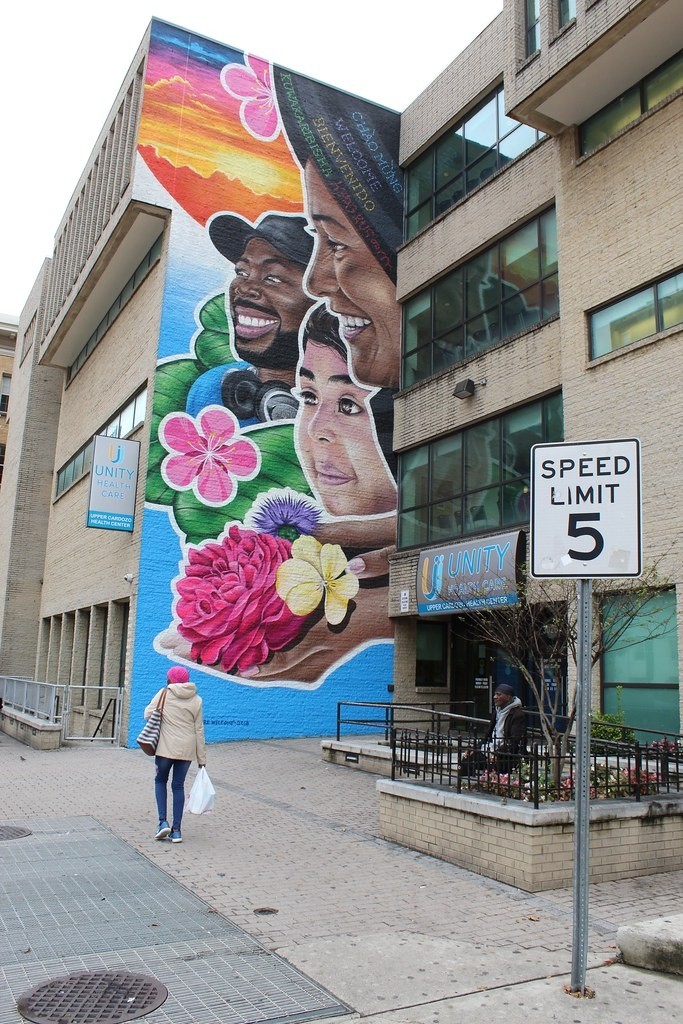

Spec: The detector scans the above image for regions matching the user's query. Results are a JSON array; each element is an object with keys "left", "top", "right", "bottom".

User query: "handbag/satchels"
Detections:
[
  {"left": 495, "top": 744, "right": 519, "bottom": 774},
  {"left": 185, "top": 766, "right": 215, "bottom": 815},
  {"left": 137, "top": 688, "right": 167, "bottom": 755}
]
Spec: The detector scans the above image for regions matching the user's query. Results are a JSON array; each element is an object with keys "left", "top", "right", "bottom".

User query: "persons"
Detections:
[
  {"left": 451, "top": 683, "right": 528, "bottom": 780},
  {"left": 141, "top": 667, "right": 206, "bottom": 843}
]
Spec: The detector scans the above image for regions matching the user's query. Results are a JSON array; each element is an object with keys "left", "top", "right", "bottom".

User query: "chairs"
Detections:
[
  {"left": 440, "top": 156, "right": 508, "bottom": 213},
  {"left": 439, "top": 322, "right": 498, "bottom": 365},
  {"left": 437, "top": 514, "right": 452, "bottom": 538},
  {"left": 454, "top": 507, "right": 471, "bottom": 532},
  {"left": 470, "top": 505, "right": 496, "bottom": 528}
]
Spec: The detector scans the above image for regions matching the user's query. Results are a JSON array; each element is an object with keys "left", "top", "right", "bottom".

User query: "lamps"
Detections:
[{"left": 451, "top": 379, "right": 489, "bottom": 400}]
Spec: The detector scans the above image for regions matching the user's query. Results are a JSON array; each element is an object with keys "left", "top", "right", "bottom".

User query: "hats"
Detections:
[
  {"left": 168, "top": 667, "right": 189, "bottom": 683},
  {"left": 495, "top": 683, "right": 515, "bottom": 696}
]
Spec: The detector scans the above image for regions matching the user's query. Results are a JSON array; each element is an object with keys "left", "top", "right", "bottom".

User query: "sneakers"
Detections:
[
  {"left": 168, "top": 826, "right": 182, "bottom": 843},
  {"left": 154, "top": 821, "right": 171, "bottom": 839}
]
